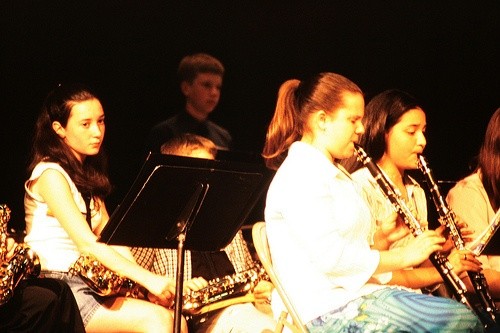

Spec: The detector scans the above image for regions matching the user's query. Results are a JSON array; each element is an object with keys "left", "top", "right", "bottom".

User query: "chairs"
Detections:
[{"left": 252, "top": 222, "right": 310, "bottom": 333}]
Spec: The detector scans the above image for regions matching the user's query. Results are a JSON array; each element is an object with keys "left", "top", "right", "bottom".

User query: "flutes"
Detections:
[
  {"left": 352, "top": 139, "right": 483, "bottom": 329},
  {"left": 412, "top": 151, "right": 500, "bottom": 323}
]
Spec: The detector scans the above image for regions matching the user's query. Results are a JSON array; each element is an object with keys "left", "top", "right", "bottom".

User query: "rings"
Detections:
[{"left": 464, "top": 254, "right": 467, "bottom": 259}]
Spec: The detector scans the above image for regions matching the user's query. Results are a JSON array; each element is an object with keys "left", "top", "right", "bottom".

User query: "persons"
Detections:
[
  {"left": 23, "top": 85, "right": 187, "bottom": 333},
  {"left": 131, "top": 132, "right": 271, "bottom": 333},
  {"left": 133, "top": 52, "right": 232, "bottom": 159},
  {"left": 336, "top": 92, "right": 500, "bottom": 300},
  {"left": 263, "top": 73, "right": 483, "bottom": 333}
]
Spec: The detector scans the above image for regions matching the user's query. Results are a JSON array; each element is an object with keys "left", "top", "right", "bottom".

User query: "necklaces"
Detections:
[{"left": 389, "top": 175, "right": 403, "bottom": 178}]
